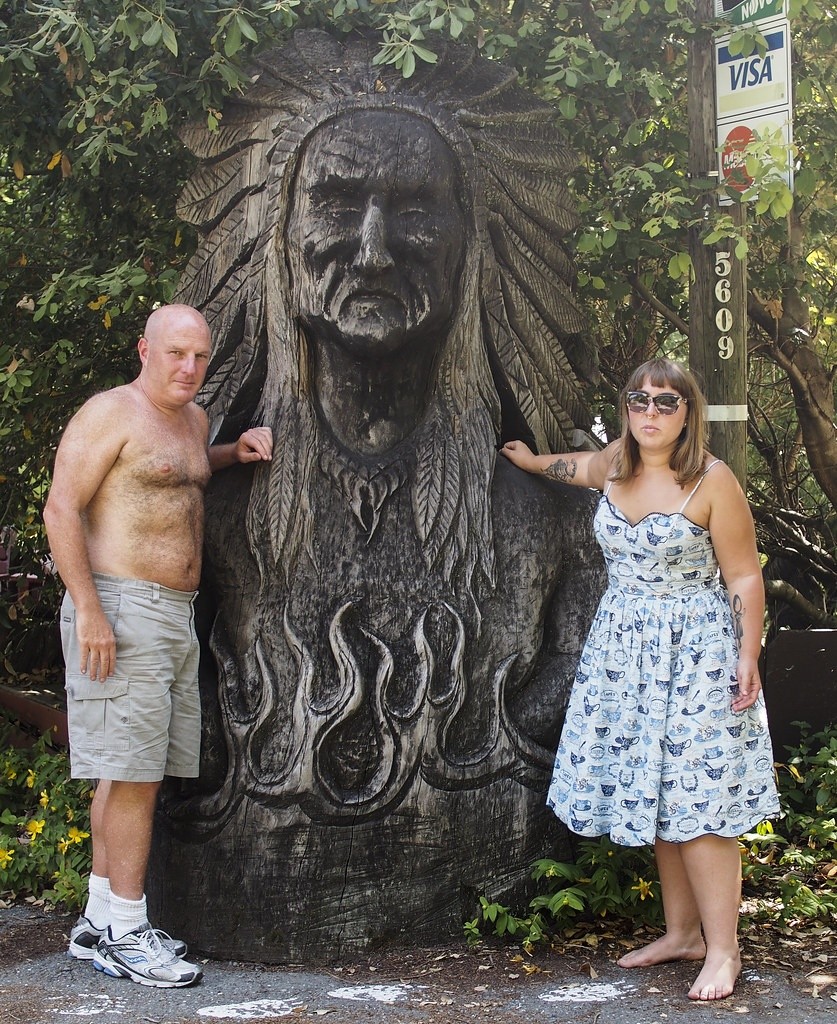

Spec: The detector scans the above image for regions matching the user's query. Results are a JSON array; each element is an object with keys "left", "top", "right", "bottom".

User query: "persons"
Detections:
[
  {"left": 500, "top": 358, "right": 781, "bottom": 1001},
  {"left": 38, "top": 305, "right": 275, "bottom": 987},
  {"left": 277, "top": 114, "right": 465, "bottom": 451}
]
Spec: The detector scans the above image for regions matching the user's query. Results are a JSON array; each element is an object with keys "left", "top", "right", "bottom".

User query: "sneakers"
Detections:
[
  {"left": 93, "top": 921, "right": 203, "bottom": 989},
  {"left": 68, "top": 915, "right": 187, "bottom": 960}
]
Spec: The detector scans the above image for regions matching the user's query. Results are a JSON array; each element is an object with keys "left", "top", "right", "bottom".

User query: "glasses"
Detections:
[{"left": 626, "top": 391, "right": 689, "bottom": 415}]
